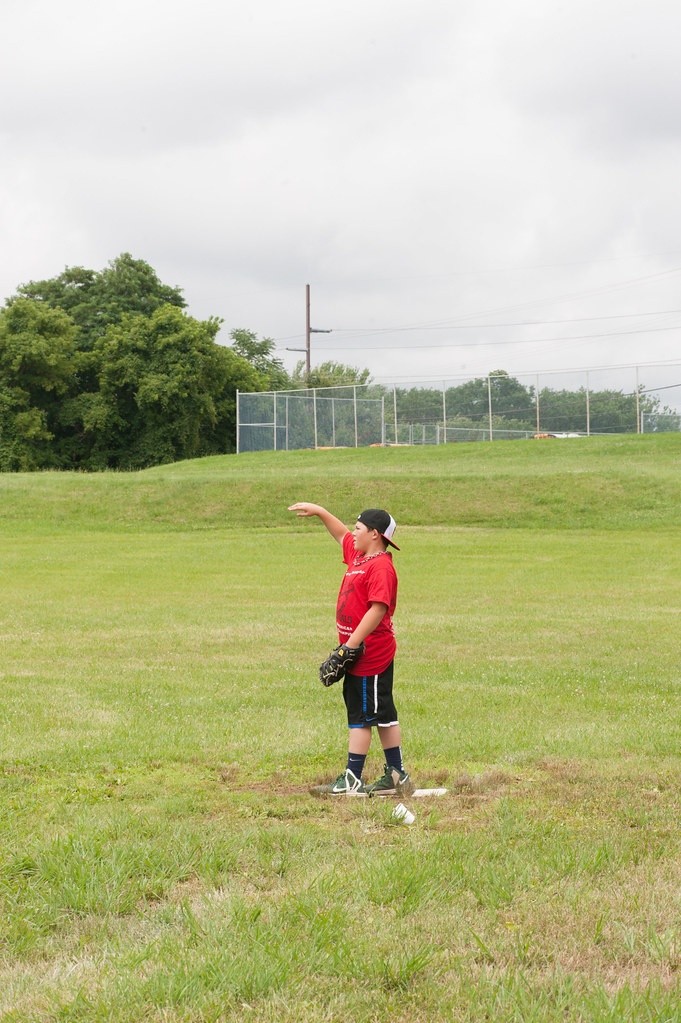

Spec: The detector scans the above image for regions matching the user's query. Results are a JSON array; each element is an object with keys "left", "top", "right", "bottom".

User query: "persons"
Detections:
[{"left": 288, "top": 501, "right": 411, "bottom": 797}]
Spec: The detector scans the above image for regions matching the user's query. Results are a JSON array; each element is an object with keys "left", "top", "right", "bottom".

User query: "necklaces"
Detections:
[{"left": 354, "top": 551, "right": 389, "bottom": 566}]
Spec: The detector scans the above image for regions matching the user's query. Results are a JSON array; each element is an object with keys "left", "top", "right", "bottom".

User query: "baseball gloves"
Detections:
[{"left": 319, "top": 643, "right": 363, "bottom": 686}]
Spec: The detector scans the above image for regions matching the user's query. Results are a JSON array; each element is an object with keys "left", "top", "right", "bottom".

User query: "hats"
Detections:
[{"left": 358, "top": 509, "right": 399, "bottom": 551}]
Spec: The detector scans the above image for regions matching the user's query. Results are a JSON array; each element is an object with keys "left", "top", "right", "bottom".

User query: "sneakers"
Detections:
[
  {"left": 314, "top": 768, "right": 367, "bottom": 795},
  {"left": 362, "top": 763, "right": 410, "bottom": 796}
]
